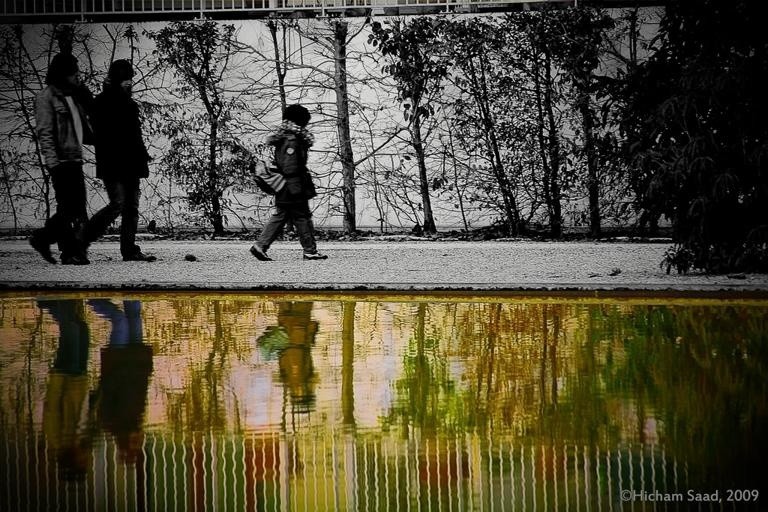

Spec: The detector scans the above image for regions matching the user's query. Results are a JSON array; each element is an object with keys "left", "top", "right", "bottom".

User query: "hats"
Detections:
[
  {"left": 109, "top": 59, "right": 134, "bottom": 83},
  {"left": 283, "top": 104, "right": 311, "bottom": 126},
  {"left": 47, "top": 52, "right": 78, "bottom": 78}
]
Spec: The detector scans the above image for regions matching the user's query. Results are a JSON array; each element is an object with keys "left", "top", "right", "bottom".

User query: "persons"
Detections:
[
  {"left": 250, "top": 104, "right": 328, "bottom": 261},
  {"left": 59, "top": 59, "right": 156, "bottom": 264},
  {"left": 38, "top": 299, "right": 97, "bottom": 480},
  {"left": 30, "top": 52, "right": 95, "bottom": 264},
  {"left": 90, "top": 298, "right": 153, "bottom": 462},
  {"left": 279, "top": 301, "right": 318, "bottom": 404}
]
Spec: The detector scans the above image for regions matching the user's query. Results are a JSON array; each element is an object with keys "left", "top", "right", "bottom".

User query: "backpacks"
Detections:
[{"left": 251, "top": 143, "right": 288, "bottom": 195}]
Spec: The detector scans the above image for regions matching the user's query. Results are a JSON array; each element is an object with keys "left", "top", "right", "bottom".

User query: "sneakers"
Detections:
[
  {"left": 250, "top": 246, "right": 271, "bottom": 261},
  {"left": 61, "top": 253, "right": 90, "bottom": 264},
  {"left": 29, "top": 235, "right": 57, "bottom": 264},
  {"left": 303, "top": 252, "right": 328, "bottom": 260},
  {"left": 123, "top": 250, "right": 157, "bottom": 261}
]
